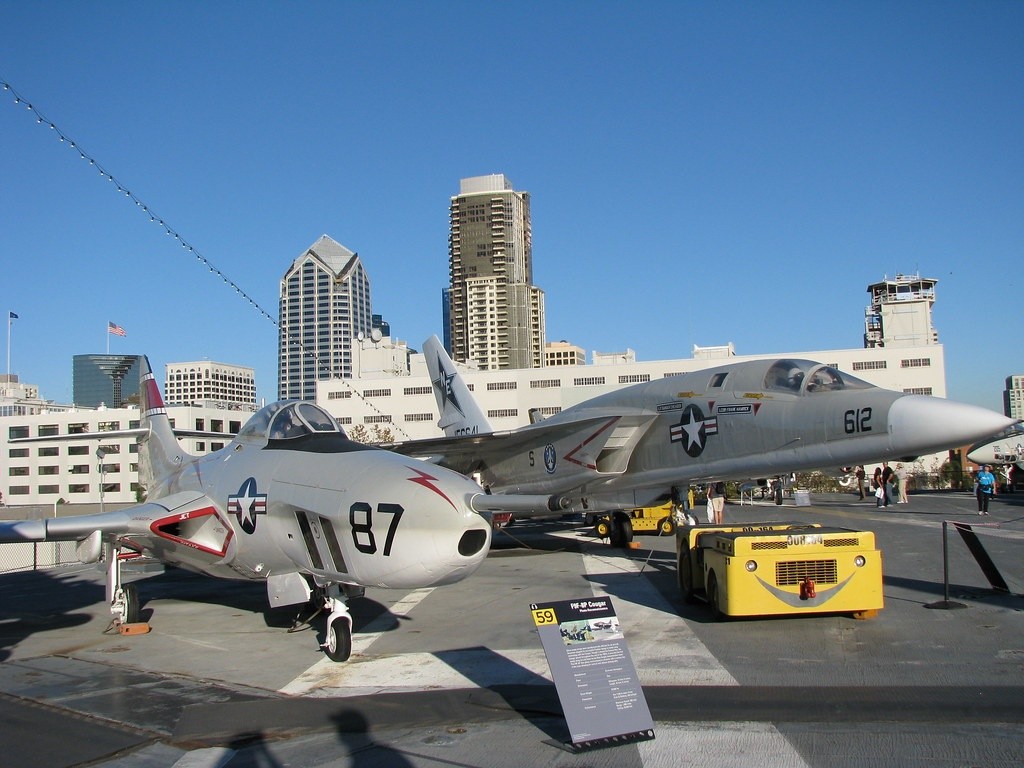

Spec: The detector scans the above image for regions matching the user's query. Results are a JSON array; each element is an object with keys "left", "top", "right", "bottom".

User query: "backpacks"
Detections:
[{"left": 711, "top": 482, "right": 727, "bottom": 494}]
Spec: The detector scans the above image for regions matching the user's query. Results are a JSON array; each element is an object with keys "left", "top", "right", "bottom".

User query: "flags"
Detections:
[
  {"left": 109, "top": 321, "right": 126, "bottom": 337},
  {"left": 10, "top": 312, "right": 18, "bottom": 318}
]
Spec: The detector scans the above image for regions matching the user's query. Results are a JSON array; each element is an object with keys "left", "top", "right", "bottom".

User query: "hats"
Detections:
[
  {"left": 983, "top": 464, "right": 993, "bottom": 470},
  {"left": 897, "top": 463, "right": 903, "bottom": 468}
]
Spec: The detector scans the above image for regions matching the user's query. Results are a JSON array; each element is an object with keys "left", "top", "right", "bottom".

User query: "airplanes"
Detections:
[{"left": 1, "top": 332, "right": 1023, "bottom": 666}]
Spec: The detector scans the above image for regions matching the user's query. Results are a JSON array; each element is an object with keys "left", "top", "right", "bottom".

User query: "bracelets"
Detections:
[{"left": 707, "top": 495, "right": 708, "bottom": 496}]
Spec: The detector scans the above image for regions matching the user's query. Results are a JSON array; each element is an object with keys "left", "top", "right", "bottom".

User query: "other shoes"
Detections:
[
  {"left": 878, "top": 505, "right": 885, "bottom": 508},
  {"left": 984, "top": 512, "right": 988, "bottom": 515},
  {"left": 856, "top": 500, "right": 864, "bottom": 503},
  {"left": 897, "top": 501, "right": 904, "bottom": 504},
  {"left": 978, "top": 511, "right": 982, "bottom": 515},
  {"left": 905, "top": 501, "right": 908, "bottom": 503}
]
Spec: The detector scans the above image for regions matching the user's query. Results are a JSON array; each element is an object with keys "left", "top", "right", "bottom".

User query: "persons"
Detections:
[
  {"left": 975, "top": 463, "right": 999, "bottom": 516},
  {"left": 767, "top": 471, "right": 797, "bottom": 498},
  {"left": 260, "top": 403, "right": 297, "bottom": 439},
  {"left": 706, "top": 481, "right": 724, "bottom": 524},
  {"left": 874, "top": 460, "right": 909, "bottom": 508},
  {"left": 789, "top": 369, "right": 814, "bottom": 393},
  {"left": 855, "top": 465, "right": 866, "bottom": 502}
]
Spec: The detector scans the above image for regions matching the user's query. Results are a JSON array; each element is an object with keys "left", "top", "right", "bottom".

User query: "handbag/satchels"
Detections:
[
  {"left": 874, "top": 487, "right": 884, "bottom": 499},
  {"left": 979, "top": 484, "right": 992, "bottom": 491}
]
